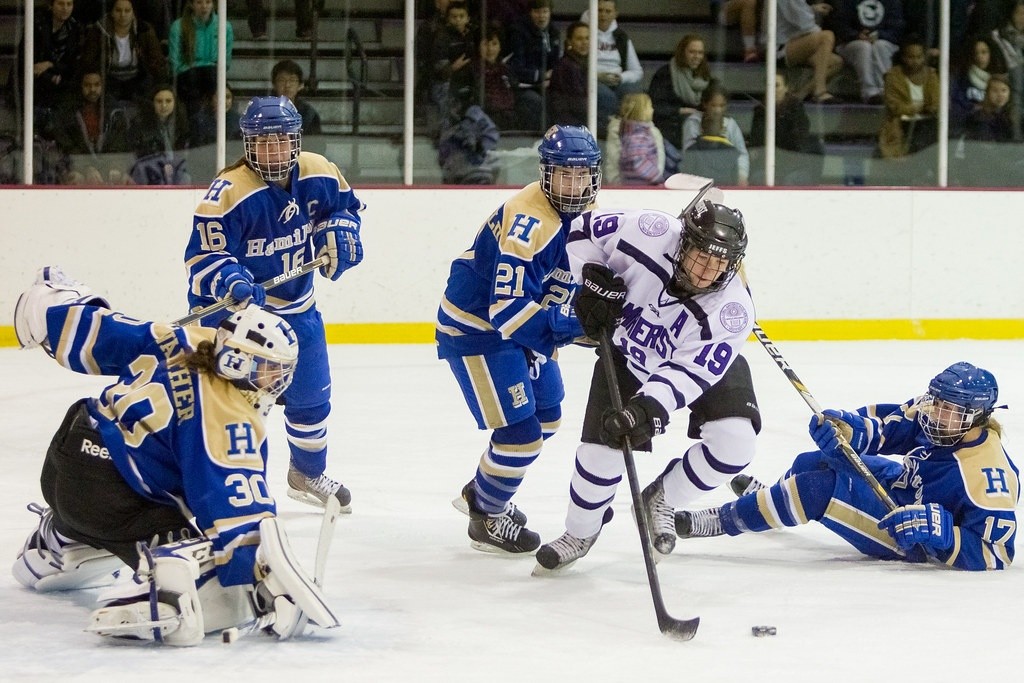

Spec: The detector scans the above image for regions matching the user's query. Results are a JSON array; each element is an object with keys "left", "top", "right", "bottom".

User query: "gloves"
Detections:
[
  {"left": 574, "top": 262, "right": 628, "bottom": 340},
  {"left": 877, "top": 504, "right": 954, "bottom": 551},
  {"left": 808, "top": 409, "right": 869, "bottom": 458},
  {"left": 599, "top": 392, "right": 670, "bottom": 448},
  {"left": 310, "top": 217, "right": 364, "bottom": 280},
  {"left": 210, "top": 264, "right": 267, "bottom": 314},
  {"left": 548, "top": 303, "right": 585, "bottom": 348}
]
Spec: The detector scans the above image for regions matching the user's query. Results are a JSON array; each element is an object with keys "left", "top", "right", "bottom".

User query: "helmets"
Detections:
[
  {"left": 213, "top": 302, "right": 299, "bottom": 400},
  {"left": 238, "top": 95, "right": 302, "bottom": 180},
  {"left": 672, "top": 200, "right": 747, "bottom": 294},
  {"left": 917, "top": 361, "right": 1009, "bottom": 446},
  {"left": 537, "top": 123, "right": 603, "bottom": 213}
]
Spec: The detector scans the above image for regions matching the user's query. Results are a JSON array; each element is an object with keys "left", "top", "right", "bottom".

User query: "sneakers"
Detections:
[
  {"left": 90, "top": 588, "right": 194, "bottom": 638},
  {"left": 467, "top": 509, "right": 541, "bottom": 557},
  {"left": 286, "top": 452, "right": 352, "bottom": 515},
  {"left": 532, "top": 506, "right": 613, "bottom": 577},
  {"left": 451, "top": 476, "right": 528, "bottom": 526},
  {"left": 727, "top": 474, "right": 771, "bottom": 498},
  {"left": 675, "top": 506, "right": 727, "bottom": 540},
  {"left": 631, "top": 458, "right": 680, "bottom": 565}
]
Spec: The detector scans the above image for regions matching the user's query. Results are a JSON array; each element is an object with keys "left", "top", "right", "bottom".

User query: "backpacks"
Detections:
[{"left": 129, "top": 155, "right": 191, "bottom": 184}]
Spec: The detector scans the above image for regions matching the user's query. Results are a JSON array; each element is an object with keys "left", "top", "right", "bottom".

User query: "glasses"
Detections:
[{"left": 276, "top": 78, "right": 300, "bottom": 86}]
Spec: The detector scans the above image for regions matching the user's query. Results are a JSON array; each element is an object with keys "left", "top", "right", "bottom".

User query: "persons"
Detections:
[
  {"left": 434, "top": 123, "right": 603, "bottom": 556},
  {"left": 269, "top": 59, "right": 321, "bottom": 136},
  {"left": 618, "top": 91, "right": 666, "bottom": 185},
  {"left": 531, "top": 200, "right": 761, "bottom": 578},
  {"left": 579, "top": 0, "right": 644, "bottom": 106},
  {"left": 681, "top": 84, "right": 750, "bottom": 187},
  {"left": 648, "top": 33, "right": 720, "bottom": 150},
  {"left": 674, "top": 361, "right": 1021, "bottom": 572},
  {"left": 0, "top": 0, "right": 1024, "bottom": 187},
  {"left": 183, "top": 95, "right": 368, "bottom": 515},
  {"left": 133, "top": 84, "right": 191, "bottom": 159},
  {"left": 878, "top": 38, "right": 940, "bottom": 158},
  {"left": 11, "top": 264, "right": 343, "bottom": 648}
]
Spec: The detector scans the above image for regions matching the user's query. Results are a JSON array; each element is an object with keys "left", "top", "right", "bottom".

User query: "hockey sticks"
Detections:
[
  {"left": 313, "top": 493, "right": 341, "bottom": 586},
  {"left": 752, "top": 321, "right": 962, "bottom": 571},
  {"left": 169, "top": 254, "right": 331, "bottom": 327},
  {"left": 597, "top": 326, "right": 700, "bottom": 642}
]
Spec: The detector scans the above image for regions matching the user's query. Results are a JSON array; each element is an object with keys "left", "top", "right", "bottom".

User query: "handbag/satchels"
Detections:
[{"left": 1, "top": 130, "right": 73, "bottom": 188}]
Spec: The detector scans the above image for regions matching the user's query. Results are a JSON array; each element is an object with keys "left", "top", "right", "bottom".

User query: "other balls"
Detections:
[{"left": 751, "top": 625, "right": 777, "bottom": 637}]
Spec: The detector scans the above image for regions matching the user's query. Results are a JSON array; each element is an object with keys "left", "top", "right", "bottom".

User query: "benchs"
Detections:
[{"left": 224, "top": 0, "right": 903, "bottom": 184}]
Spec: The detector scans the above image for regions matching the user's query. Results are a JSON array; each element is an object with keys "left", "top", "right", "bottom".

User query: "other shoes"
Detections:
[
  {"left": 251, "top": 23, "right": 270, "bottom": 41},
  {"left": 296, "top": 25, "right": 313, "bottom": 41}
]
